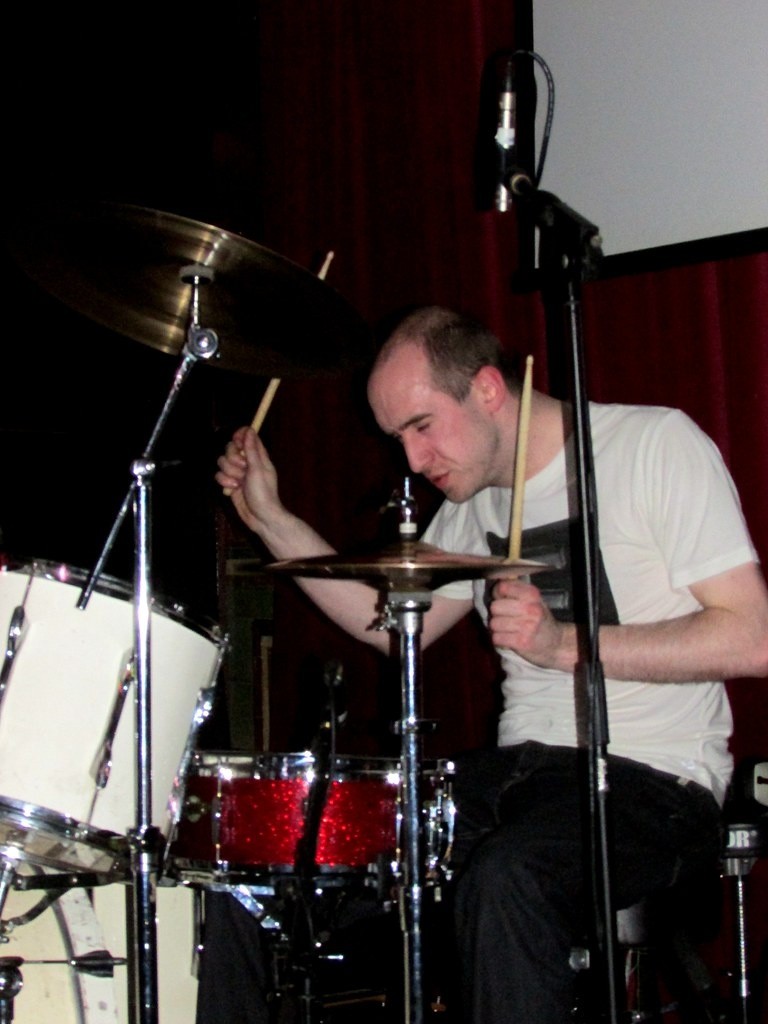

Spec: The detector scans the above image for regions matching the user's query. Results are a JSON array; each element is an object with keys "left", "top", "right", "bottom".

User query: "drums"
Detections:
[
  {"left": 0, "top": 864, "right": 206, "bottom": 1023},
  {"left": 2, "top": 556, "right": 233, "bottom": 884},
  {"left": 163, "top": 748, "right": 459, "bottom": 900}
]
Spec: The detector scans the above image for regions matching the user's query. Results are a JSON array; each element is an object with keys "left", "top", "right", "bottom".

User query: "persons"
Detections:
[{"left": 215, "top": 309, "right": 768, "bottom": 1024}]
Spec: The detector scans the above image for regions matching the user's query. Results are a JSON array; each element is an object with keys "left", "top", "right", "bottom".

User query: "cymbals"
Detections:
[
  {"left": 262, "top": 534, "right": 556, "bottom": 593},
  {"left": 37, "top": 193, "right": 369, "bottom": 379}
]
